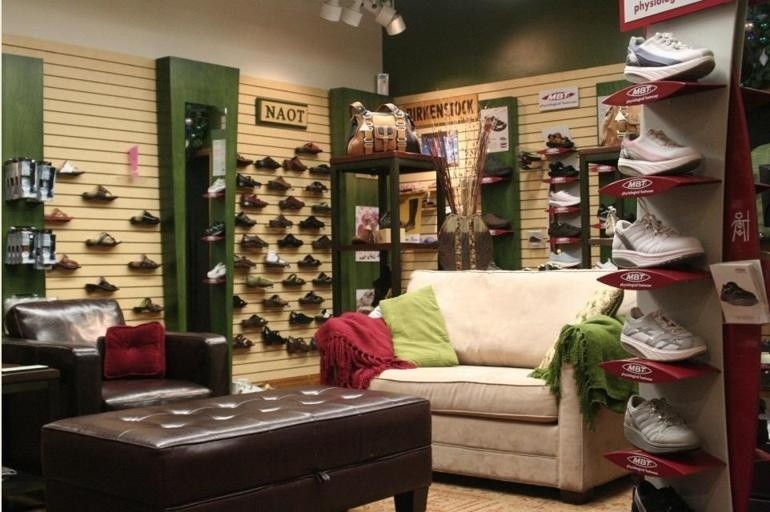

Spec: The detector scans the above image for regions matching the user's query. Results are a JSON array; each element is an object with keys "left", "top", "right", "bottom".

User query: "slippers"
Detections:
[
  {"left": 232, "top": 290, "right": 331, "bottom": 354},
  {"left": 43, "top": 208, "right": 70, "bottom": 221},
  {"left": 236, "top": 143, "right": 330, "bottom": 192},
  {"left": 128, "top": 256, "right": 160, "bottom": 270},
  {"left": 83, "top": 185, "right": 118, "bottom": 200},
  {"left": 234, "top": 193, "right": 332, "bottom": 249},
  {"left": 133, "top": 298, "right": 164, "bottom": 313},
  {"left": 56, "top": 160, "right": 84, "bottom": 175},
  {"left": 234, "top": 254, "right": 332, "bottom": 288},
  {"left": 85, "top": 280, "right": 119, "bottom": 292},
  {"left": 131, "top": 211, "right": 159, "bottom": 224},
  {"left": 57, "top": 254, "right": 82, "bottom": 270},
  {"left": 86, "top": 232, "right": 121, "bottom": 246}
]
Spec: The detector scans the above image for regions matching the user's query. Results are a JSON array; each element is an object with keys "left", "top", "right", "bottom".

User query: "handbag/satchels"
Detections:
[{"left": 345, "top": 100, "right": 423, "bottom": 156}]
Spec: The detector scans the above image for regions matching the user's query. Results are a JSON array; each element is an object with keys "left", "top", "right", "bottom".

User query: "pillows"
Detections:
[
  {"left": 102, "top": 321, "right": 167, "bottom": 379},
  {"left": 378, "top": 285, "right": 460, "bottom": 368},
  {"left": 536, "top": 285, "right": 624, "bottom": 375}
]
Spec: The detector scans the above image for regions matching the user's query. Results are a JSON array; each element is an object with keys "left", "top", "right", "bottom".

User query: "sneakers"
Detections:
[
  {"left": 617, "top": 128, "right": 704, "bottom": 177},
  {"left": 548, "top": 190, "right": 581, "bottom": 206},
  {"left": 485, "top": 115, "right": 506, "bottom": 131},
  {"left": 611, "top": 212, "right": 705, "bottom": 269},
  {"left": 632, "top": 479, "right": 695, "bottom": 512},
  {"left": 485, "top": 159, "right": 513, "bottom": 177},
  {"left": 204, "top": 178, "right": 226, "bottom": 279},
  {"left": 619, "top": 307, "right": 708, "bottom": 362},
  {"left": 624, "top": 394, "right": 703, "bottom": 455},
  {"left": 624, "top": 31, "right": 715, "bottom": 84},
  {"left": 482, "top": 211, "right": 510, "bottom": 229},
  {"left": 548, "top": 222, "right": 581, "bottom": 237},
  {"left": 721, "top": 282, "right": 759, "bottom": 306},
  {"left": 549, "top": 251, "right": 583, "bottom": 268}
]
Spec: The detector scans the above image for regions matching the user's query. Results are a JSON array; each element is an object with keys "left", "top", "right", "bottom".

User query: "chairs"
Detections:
[{"left": 5, "top": 299, "right": 229, "bottom": 418}]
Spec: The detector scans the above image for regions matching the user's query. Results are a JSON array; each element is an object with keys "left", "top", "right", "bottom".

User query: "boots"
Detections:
[{"left": 404, "top": 198, "right": 418, "bottom": 232}]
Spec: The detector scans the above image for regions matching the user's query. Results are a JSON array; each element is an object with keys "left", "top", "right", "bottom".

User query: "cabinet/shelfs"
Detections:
[
  {"left": 479, "top": 176, "right": 514, "bottom": 236},
  {"left": 590, "top": 79, "right": 728, "bottom": 480},
  {"left": 329, "top": 150, "right": 445, "bottom": 316},
  {"left": 537, "top": 146, "right": 583, "bottom": 247},
  {"left": 578, "top": 146, "right": 636, "bottom": 268}
]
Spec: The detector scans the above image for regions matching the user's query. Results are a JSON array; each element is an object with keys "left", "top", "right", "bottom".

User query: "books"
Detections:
[{"left": 709, "top": 258, "right": 769, "bottom": 326}]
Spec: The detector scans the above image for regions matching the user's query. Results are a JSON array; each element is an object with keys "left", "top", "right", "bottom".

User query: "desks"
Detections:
[{"left": 2, "top": 362, "right": 61, "bottom": 511}]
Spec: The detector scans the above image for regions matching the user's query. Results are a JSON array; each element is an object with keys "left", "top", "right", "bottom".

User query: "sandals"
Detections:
[
  {"left": 546, "top": 133, "right": 574, "bottom": 147},
  {"left": 548, "top": 161, "right": 579, "bottom": 177}
]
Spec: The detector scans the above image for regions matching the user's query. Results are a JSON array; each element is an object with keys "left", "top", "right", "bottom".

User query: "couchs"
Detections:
[{"left": 319, "top": 268, "right": 637, "bottom": 503}]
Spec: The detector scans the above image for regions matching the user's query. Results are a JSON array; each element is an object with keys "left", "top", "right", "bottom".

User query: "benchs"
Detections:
[{"left": 41, "top": 386, "right": 432, "bottom": 511}]
